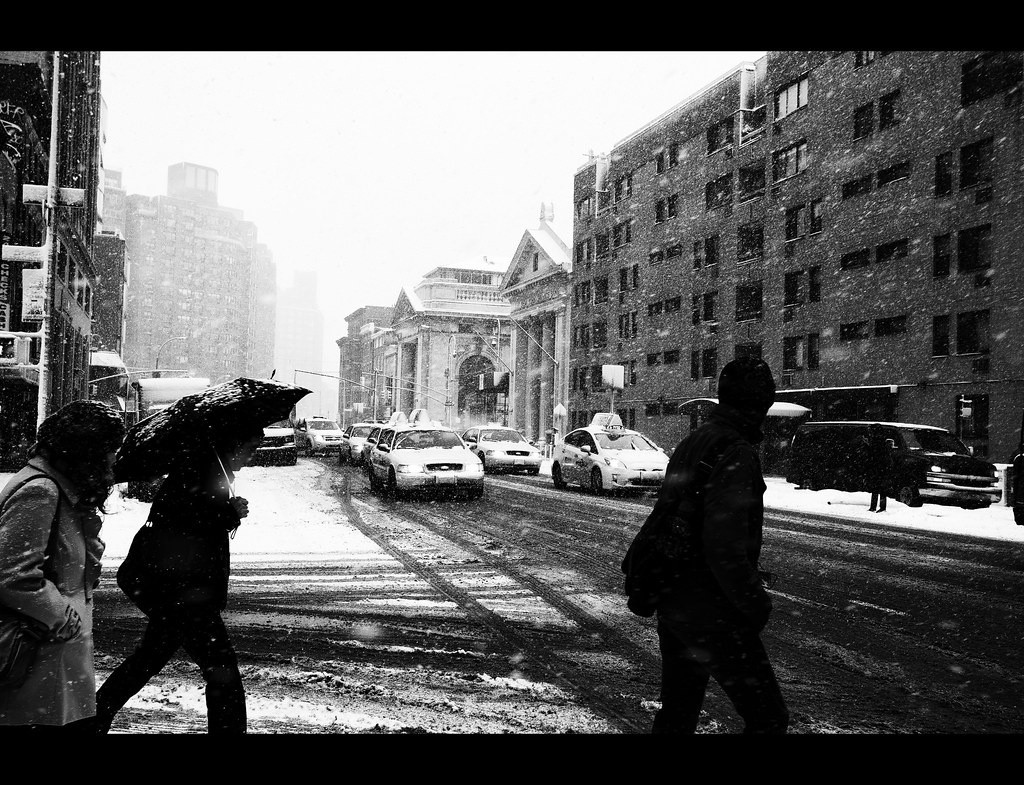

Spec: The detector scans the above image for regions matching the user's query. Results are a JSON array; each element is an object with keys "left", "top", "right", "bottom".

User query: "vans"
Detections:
[{"left": 785, "top": 419, "right": 1003, "bottom": 510}]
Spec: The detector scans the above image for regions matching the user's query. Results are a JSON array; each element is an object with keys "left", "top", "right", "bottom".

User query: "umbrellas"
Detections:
[{"left": 112, "top": 376, "right": 314, "bottom": 500}]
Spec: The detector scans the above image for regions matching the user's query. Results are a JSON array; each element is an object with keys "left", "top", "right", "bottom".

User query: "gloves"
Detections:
[{"left": 744, "top": 580, "right": 773, "bottom": 632}]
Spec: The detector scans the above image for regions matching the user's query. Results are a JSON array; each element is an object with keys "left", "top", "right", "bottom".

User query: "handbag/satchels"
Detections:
[
  {"left": 116, "top": 523, "right": 203, "bottom": 634},
  {"left": 0, "top": 473, "right": 63, "bottom": 692},
  {"left": 622, "top": 434, "right": 736, "bottom": 620}
]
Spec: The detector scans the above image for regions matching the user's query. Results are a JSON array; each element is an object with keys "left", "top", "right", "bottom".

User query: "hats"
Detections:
[
  {"left": 38, "top": 397, "right": 127, "bottom": 467},
  {"left": 718, "top": 357, "right": 777, "bottom": 420}
]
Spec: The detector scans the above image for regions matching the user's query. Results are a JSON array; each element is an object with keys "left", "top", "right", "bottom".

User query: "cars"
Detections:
[
  {"left": 248, "top": 414, "right": 298, "bottom": 466},
  {"left": 360, "top": 422, "right": 484, "bottom": 500},
  {"left": 338, "top": 423, "right": 376, "bottom": 463},
  {"left": 549, "top": 424, "right": 671, "bottom": 497},
  {"left": 293, "top": 415, "right": 344, "bottom": 458},
  {"left": 461, "top": 422, "right": 542, "bottom": 474}
]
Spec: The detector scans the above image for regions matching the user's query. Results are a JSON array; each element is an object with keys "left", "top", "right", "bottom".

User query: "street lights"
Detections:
[
  {"left": 421, "top": 323, "right": 458, "bottom": 425},
  {"left": 152, "top": 335, "right": 189, "bottom": 378}
]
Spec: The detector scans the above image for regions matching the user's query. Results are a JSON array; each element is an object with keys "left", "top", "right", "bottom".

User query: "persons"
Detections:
[
  {"left": 861, "top": 424, "right": 889, "bottom": 512},
  {"left": 94, "top": 414, "right": 264, "bottom": 734},
  {"left": 649, "top": 357, "right": 790, "bottom": 733},
  {"left": 0, "top": 400, "right": 128, "bottom": 731}
]
